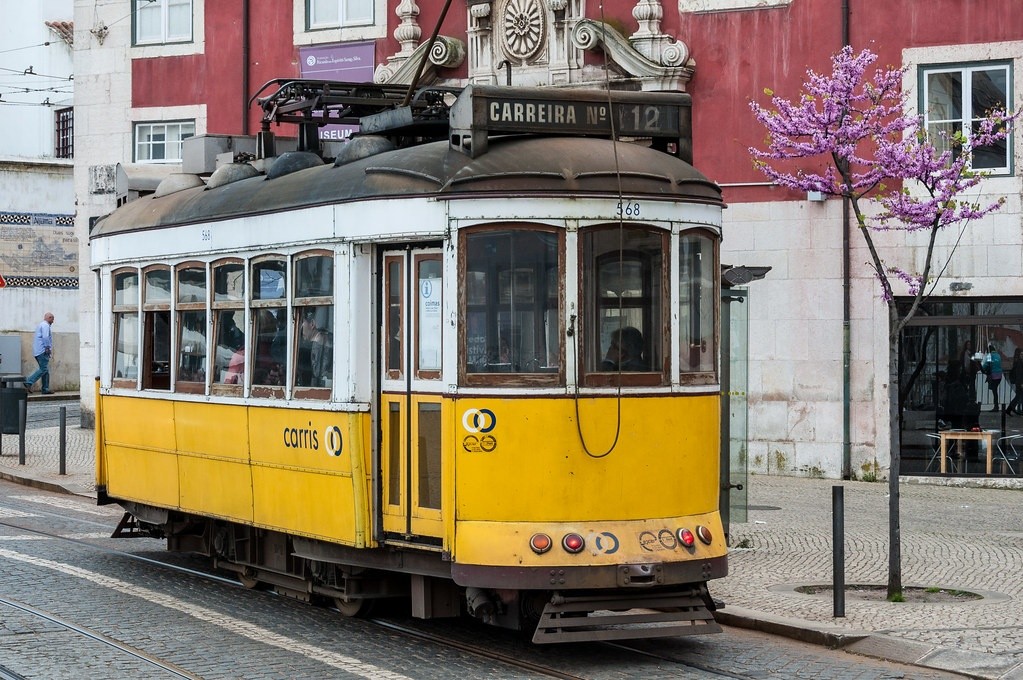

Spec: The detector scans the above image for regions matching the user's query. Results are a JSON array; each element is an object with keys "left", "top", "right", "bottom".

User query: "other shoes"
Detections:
[
  {"left": 22, "top": 379, "right": 33, "bottom": 393},
  {"left": 42, "top": 390, "right": 53, "bottom": 394}
]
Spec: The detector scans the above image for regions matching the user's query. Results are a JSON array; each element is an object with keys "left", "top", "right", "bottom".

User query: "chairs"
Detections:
[
  {"left": 992, "top": 430, "right": 1023, "bottom": 474},
  {"left": 922, "top": 429, "right": 968, "bottom": 474}
]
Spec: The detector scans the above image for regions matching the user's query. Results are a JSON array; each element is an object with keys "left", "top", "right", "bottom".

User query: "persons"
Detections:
[
  {"left": 981, "top": 346, "right": 1002, "bottom": 412},
  {"left": 1006, "top": 348, "right": 1023, "bottom": 416},
  {"left": 931, "top": 371, "right": 948, "bottom": 450},
  {"left": 601, "top": 325, "right": 645, "bottom": 372},
  {"left": 945, "top": 367, "right": 982, "bottom": 463},
  {"left": 226, "top": 310, "right": 278, "bottom": 385},
  {"left": 302, "top": 309, "right": 333, "bottom": 386},
  {"left": 961, "top": 339, "right": 978, "bottom": 384},
  {"left": 23, "top": 313, "right": 54, "bottom": 395}
]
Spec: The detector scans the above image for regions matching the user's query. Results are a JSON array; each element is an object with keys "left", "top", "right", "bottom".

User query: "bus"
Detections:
[{"left": 87, "top": 71, "right": 731, "bottom": 645}]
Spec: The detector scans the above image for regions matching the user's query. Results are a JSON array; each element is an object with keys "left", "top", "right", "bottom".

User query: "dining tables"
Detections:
[{"left": 939, "top": 429, "right": 1002, "bottom": 474}]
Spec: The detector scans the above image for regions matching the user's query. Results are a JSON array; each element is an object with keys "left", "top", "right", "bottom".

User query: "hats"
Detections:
[{"left": 233, "top": 310, "right": 277, "bottom": 341}]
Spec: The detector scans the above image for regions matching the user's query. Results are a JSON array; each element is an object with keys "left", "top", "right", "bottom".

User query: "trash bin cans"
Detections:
[{"left": 0, "top": 374, "right": 29, "bottom": 435}]
